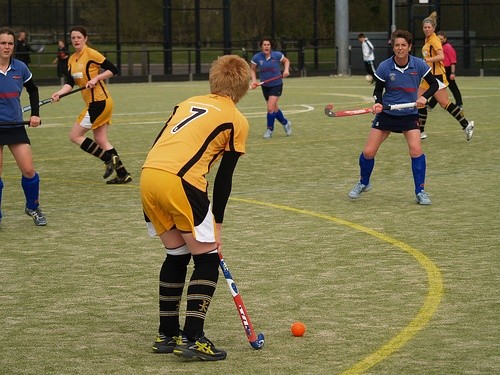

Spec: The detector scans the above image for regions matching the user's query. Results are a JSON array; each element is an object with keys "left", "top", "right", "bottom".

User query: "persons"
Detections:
[
  {"left": 137, "top": 55, "right": 250, "bottom": 361},
  {"left": 418, "top": 11, "right": 475, "bottom": 141},
  {"left": 52, "top": 38, "right": 70, "bottom": 89},
  {"left": 250, "top": 37, "right": 292, "bottom": 138},
  {"left": 349, "top": 29, "right": 439, "bottom": 205},
  {"left": 358, "top": 33, "right": 376, "bottom": 76},
  {"left": 13, "top": 30, "right": 39, "bottom": 66},
  {"left": 0, "top": 26, "right": 48, "bottom": 226},
  {"left": 50, "top": 25, "right": 133, "bottom": 185},
  {"left": 426, "top": 31, "right": 463, "bottom": 112}
]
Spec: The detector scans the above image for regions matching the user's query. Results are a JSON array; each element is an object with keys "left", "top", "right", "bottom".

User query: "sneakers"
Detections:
[
  {"left": 174, "top": 331, "right": 227, "bottom": 361},
  {"left": 152, "top": 330, "right": 182, "bottom": 353},
  {"left": 25, "top": 206, "right": 48, "bottom": 226}
]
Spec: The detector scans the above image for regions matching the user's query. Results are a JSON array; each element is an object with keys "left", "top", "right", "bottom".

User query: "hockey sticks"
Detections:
[
  {"left": 217, "top": 251, "right": 265, "bottom": 349},
  {"left": 22, "top": 83, "right": 97, "bottom": 113},
  {"left": 0, "top": 120, "right": 41, "bottom": 125},
  {"left": 248, "top": 75, "right": 284, "bottom": 89},
  {"left": 324, "top": 101, "right": 429, "bottom": 117}
]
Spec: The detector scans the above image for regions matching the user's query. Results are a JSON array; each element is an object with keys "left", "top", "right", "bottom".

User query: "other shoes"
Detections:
[
  {"left": 416, "top": 190, "right": 431, "bottom": 205},
  {"left": 103, "top": 156, "right": 118, "bottom": 178},
  {"left": 462, "top": 121, "right": 474, "bottom": 141},
  {"left": 262, "top": 129, "right": 273, "bottom": 138},
  {"left": 420, "top": 132, "right": 427, "bottom": 139},
  {"left": 349, "top": 179, "right": 372, "bottom": 198},
  {"left": 107, "top": 173, "right": 132, "bottom": 184},
  {"left": 283, "top": 121, "right": 292, "bottom": 136}
]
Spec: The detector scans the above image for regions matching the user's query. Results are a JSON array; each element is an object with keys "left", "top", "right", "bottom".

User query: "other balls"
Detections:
[{"left": 291, "top": 322, "right": 306, "bottom": 336}]
